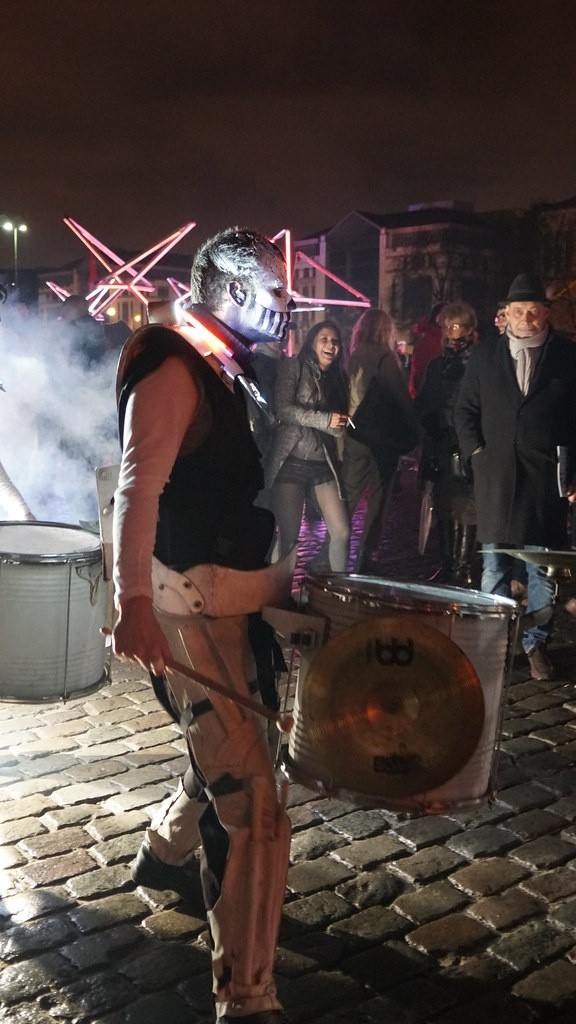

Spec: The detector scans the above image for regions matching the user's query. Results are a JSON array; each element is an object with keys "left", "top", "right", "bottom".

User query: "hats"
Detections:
[{"left": 506, "top": 272, "right": 547, "bottom": 300}]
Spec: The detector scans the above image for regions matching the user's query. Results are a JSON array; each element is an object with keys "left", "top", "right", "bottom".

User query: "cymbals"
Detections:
[{"left": 477, "top": 549, "right": 576, "bottom": 577}]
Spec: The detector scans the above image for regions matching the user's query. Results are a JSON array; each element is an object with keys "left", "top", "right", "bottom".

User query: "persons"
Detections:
[
  {"left": 341, "top": 300, "right": 508, "bottom": 591},
  {"left": 110, "top": 225, "right": 297, "bottom": 1024},
  {"left": 5, "top": 295, "right": 110, "bottom": 387},
  {"left": 450, "top": 270, "right": 576, "bottom": 683},
  {"left": 266, "top": 320, "right": 351, "bottom": 610},
  {"left": 245, "top": 316, "right": 296, "bottom": 565}
]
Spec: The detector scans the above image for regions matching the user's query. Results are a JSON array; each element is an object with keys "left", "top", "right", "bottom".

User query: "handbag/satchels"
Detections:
[
  {"left": 345, "top": 351, "right": 416, "bottom": 456},
  {"left": 420, "top": 449, "right": 472, "bottom": 483}
]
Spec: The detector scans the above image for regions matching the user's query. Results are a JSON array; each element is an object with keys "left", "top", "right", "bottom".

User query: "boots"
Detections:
[
  {"left": 427, "top": 519, "right": 464, "bottom": 584},
  {"left": 446, "top": 523, "right": 477, "bottom": 588}
]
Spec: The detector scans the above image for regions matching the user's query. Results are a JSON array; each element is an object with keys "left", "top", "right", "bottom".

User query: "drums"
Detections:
[
  {"left": 277, "top": 565, "right": 522, "bottom": 815},
  {"left": 0, "top": 519, "right": 114, "bottom": 703}
]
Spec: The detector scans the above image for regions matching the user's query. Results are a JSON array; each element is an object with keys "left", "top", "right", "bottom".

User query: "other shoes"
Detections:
[
  {"left": 215, "top": 1008, "right": 297, "bottom": 1024},
  {"left": 526, "top": 640, "right": 556, "bottom": 681},
  {"left": 564, "top": 597, "right": 576, "bottom": 617},
  {"left": 132, "top": 845, "right": 204, "bottom": 906}
]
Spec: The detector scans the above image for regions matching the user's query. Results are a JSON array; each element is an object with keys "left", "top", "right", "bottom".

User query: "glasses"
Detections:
[
  {"left": 444, "top": 323, "right": 464, "bottom": 330},
  {"left": 494, "top": 314, "right": 504, "bottom": 323}
]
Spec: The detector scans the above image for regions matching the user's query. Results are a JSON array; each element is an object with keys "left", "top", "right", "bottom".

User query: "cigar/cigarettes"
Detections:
[{"left": 348, "top": 418, "right": 356, "bottom": 429}]
